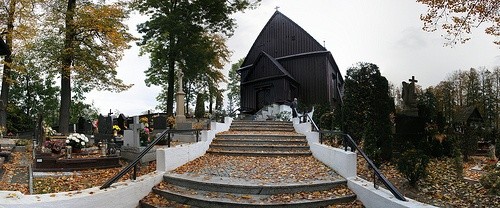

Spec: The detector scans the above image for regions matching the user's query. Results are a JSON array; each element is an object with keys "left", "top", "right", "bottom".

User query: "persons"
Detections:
[{"left": 290, "top": 98, "right": 298, "bottom": 119}]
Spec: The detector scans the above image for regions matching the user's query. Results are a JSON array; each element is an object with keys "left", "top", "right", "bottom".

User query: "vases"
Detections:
[
  {"left": 112, "top": 130, "right": 119, "bottom": 136},
  {"left": 51, "top": 148, "right": 62, "bottom": 156},
  {"left": 71, "top": 146, "right": 82, "bottom": 154}
]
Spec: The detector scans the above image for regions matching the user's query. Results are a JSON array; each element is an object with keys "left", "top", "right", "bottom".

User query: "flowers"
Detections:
[
  {"left": 65, "top": 132, "right": 90, "bottom": 149},
  {"left": 112, "top": 125, "right": 121, "bottom": 132},
  {"left": 44, "top": 139, "right": 64, "bottom": 152}
]
